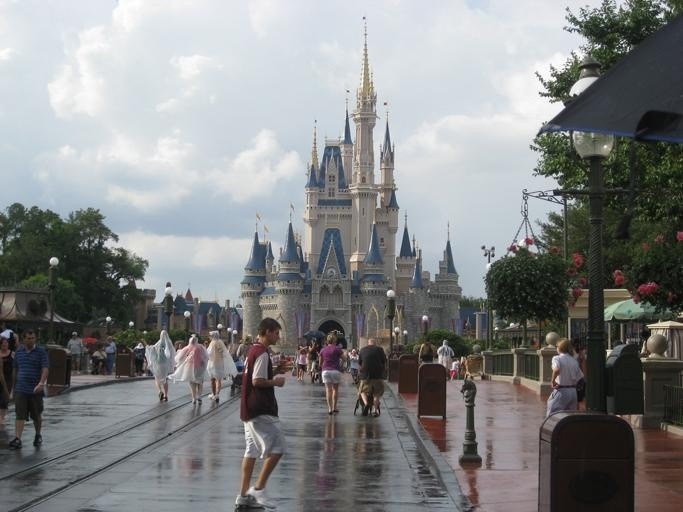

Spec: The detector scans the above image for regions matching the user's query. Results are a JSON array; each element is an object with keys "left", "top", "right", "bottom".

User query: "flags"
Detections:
[
  {"left": 361, "top": 16, "right": 367, "bottom": 20},
  {"left": 289, "top": 202, "right": 295, "bottom": 212},
  {"left": 254, "top": 210, "right": 261, "bottom": 219},
  {"left": 383, "top": 102, "right": 388, "bottom": 108},
  {"left": 264, "top": 225, "right": 270, "bottom": 233},
  {"left": 345, "top": 89, "right": 349, "bottom": 94}
]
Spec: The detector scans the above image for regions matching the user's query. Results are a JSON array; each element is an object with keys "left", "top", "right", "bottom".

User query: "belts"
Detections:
[{"left": 560, "top": 385, "right": 575, "bottom": 388}]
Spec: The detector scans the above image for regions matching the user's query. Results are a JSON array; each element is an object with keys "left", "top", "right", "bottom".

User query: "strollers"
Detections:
[{"left": 231, "top": 361, "right": 244, "bottom": 389}]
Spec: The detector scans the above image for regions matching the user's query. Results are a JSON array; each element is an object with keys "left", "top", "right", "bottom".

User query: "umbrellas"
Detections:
[
  {"left": 533, "top": 12, "right": 682, "bottom": 150},
  {"left": 603, "top": 296, "right": 675, "bottom": 322}
]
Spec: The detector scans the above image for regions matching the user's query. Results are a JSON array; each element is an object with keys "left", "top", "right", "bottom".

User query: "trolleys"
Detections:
[{"left": 463, "top": 355, "right": 484, "bottom": 381}]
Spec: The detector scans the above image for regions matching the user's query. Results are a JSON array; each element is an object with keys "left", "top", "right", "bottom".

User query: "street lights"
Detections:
[
  {"left": 481, "top": 238, "right": 529, "bottom": 349},
  {"left": 421, "top": 315, "right": 429, "bottom": 344},
  {"left": 217, "top": 323, "right": 238, "bottom": 344},
  {"left": 163, "top": 286, "right": 174, "bottom": 335},
  {"left": 183, "top": 311, "right": 191, "bottom": 344},
  {"left": 392, "top": 326, "right": 408, "bottom": 345},
  {"left": 106, "top": 315, "right": 147, "bottom": 339},
  {"left": 553, "top": 52, "right": 639, "bottom": 413},
  {"left": 556, "top": 173, "right": 571, "bottom": 261},
  {"left": 47, "top": 257, "right": 60, "bottom": 343},
  {"left": 386, "top": 289, "right": 396, "bottom": 355}
]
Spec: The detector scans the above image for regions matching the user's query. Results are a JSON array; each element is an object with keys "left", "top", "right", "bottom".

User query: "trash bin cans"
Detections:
[
  {"left": 537, "top": 410, "right": 635, "bottom": 511},
  {"left": 398, "top": 354, "right": 419, "bottom": 394},
  {"left": 46, "top": 347, "right": 72, "bottom": 388},
  {"left": 115, "top": 342, "right": 136, "bottom": 377},
  {"left": 417, "top": 363, "right": 447, "bottom": 419},
  {"left": 389, "top": 352, "right": 402, "bottom": 382},
  {"left": 605, "top": 344, "right": 644, "bottom": 416}
]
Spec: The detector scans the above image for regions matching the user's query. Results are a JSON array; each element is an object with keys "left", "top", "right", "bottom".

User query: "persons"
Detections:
[
  {"left": 66, "top": 332, "right": 117, "bottom": 375},
  {"left": 418, "top": 340, "right": 465, "bottom": 380},
  {"left": 357, "top": 339, "right": 387, "bottom": 416},
  {"left": 132, "top": 330, "right": 255, "bottom": 405},
  {"left": 294, "top": 335, "right": 359, "bottom": 385},
  {"left": 319, "top": 334, "right": 347, "bottom": 415},
  {"left": 233, "top": 315, "right": 287, "bottom": 512},
  {"left": 547, "top": 337, "right": 584, "bottom": 415},
  {"left": 1, "top": 329, "right": 49, "bottom": 448}
]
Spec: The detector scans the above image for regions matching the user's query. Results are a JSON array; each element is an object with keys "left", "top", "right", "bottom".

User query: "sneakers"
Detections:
[
  {"left": 10, "top": 437, "right": 22, "bottom": 448},
  {"left": 34, "top": 435, "right": 42, "bottom": 446},
  {"left": 159, "top": 392, "right": 167, "bottom": 400},
  {"left": 236, "top": 486, "right": 275, "bottom": 509}
]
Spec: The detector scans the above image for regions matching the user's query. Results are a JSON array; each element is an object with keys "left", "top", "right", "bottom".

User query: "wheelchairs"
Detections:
[{"left": 353, "top": 394, "right": 381, "bottom": 416}]
